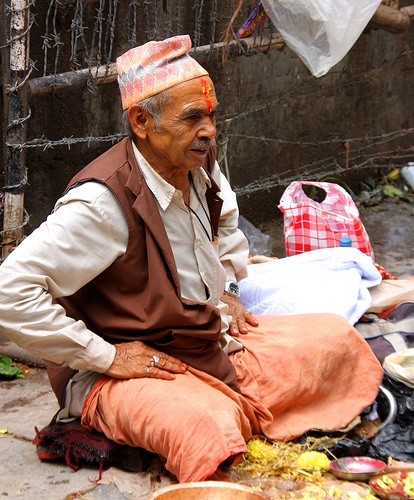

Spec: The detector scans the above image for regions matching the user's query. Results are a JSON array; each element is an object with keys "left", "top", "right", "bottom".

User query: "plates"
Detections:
[{"left": 331, "top": 458, "right": 385, "bottom": 480}]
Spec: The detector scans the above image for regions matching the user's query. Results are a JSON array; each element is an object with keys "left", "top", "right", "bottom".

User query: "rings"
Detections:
[
  {"left": 153, "top": 355, "right": 159, "bottom": 364},
  {"left": 146, "top": 368, "right": 151, "bottom": 378},
  {"left": 149, "top": 361, "right": 155, "bottom": 367}
]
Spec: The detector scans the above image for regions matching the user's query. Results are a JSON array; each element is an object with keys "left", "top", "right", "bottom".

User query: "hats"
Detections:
[{"left": 116, "top": 34, "right": 209, "bottom": 110}]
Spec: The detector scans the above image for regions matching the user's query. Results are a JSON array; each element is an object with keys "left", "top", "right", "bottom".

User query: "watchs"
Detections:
[{"left": 225, "top": 280, "right": 240, "bottom": 296}]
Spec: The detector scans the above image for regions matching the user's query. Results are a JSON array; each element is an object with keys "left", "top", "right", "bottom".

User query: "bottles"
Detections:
[{"left": 339, "top": 237, "right": 352, "bottom": 246}]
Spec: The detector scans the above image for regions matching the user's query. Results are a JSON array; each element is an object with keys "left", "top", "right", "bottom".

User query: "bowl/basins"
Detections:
[{"left": 148, "top": 482, "right": 272, "bottom": 500}]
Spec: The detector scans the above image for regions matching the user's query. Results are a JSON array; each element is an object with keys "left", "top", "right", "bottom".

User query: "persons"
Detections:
[{"left": 0, "top": 34, "right": 383, "bottom": 485}]
